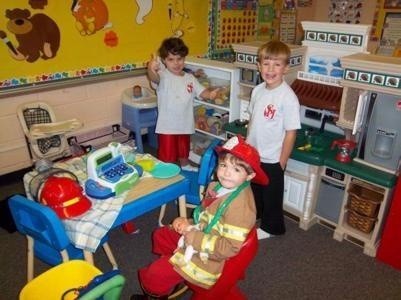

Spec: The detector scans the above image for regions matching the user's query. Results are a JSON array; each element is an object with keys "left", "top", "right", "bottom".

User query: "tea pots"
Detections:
[{"left": 331, "top": 139, "right": 356, "bottom": 164}]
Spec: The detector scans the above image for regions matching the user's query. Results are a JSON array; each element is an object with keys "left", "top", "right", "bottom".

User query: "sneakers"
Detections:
[{"left": 255, "top": 228, "right": 278, "bottom": 240}]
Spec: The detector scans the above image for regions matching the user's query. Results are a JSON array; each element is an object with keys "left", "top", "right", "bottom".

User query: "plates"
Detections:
[{"left": 152, "top": 163, "right": 181, "bottom": 179}]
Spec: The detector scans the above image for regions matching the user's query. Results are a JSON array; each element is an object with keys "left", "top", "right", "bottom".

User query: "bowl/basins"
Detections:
[{"left": 236, "top": 119, "right": 248, "bottom": 126}]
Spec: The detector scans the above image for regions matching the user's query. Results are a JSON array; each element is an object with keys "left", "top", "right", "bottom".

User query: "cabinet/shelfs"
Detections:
[
  {"left": 338, "top": 178, "right": 388, "bottom": 246},
  {"left": 282, "top": 174, "right": 308, "bottom": 213},
  {"left": 156, "top": 55, "right": 241, "bottom": 166}
]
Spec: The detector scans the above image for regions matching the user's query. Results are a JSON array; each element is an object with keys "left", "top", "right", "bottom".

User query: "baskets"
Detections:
[
  {"left": 348, "top": 186, "right": 383, "bottom": 217},
  {"left": 347, "top": 209, "right": 375, "bottom": 233}
]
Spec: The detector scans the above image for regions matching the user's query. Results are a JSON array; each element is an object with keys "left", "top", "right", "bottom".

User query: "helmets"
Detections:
[{"left": 41, "top": 176, "right": 91, "bottom": 219}]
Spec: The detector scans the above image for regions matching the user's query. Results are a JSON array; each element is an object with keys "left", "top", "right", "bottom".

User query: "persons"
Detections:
[
  {"left": 129, "top": 134, "right": 270, "bottom": 300},
  {"left": 145, "top": 38, "right": 221, "bottom": 167},
  {"left": 243, "top": 41, "right": 302, "bottom": 240}
]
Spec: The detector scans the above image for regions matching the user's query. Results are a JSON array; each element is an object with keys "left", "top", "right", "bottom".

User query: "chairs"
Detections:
[
  {"left": 167, "top": 224, "right": 260, "bottom": 300},
  {"left": 158, "top": 138, "right": 222, "bottom": 228},
  {"left": 9, "top": 194, "right": 118, "bottom": 283},
  {"left": 19, "top": 260, "right": 126, "bottom": 300},
  {"left": 121, "top": 86, "right": 159, "bottom": 154}
]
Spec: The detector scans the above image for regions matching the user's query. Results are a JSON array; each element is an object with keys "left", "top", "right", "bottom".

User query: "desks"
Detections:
[{"left": 23, "top": 143, "right": 191, "bottom": 267}]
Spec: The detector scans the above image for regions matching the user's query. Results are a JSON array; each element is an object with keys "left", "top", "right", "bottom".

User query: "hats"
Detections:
[{"left": 215, "top": 135, "right": 269, "bottom": 185}]
